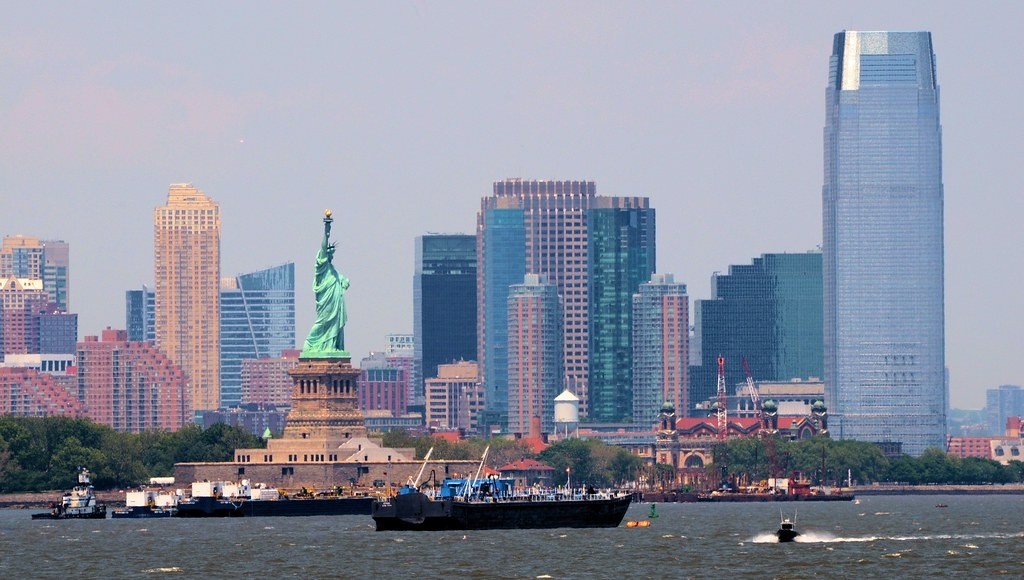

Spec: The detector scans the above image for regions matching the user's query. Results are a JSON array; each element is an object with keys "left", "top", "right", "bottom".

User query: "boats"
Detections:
[
  {"left": 113, "top": 476, "right": 447, "bottom": 518},
  {"left": 372, "top": 480, "right": 632, "bottom": 531},
  {"left": 31, "top": 466, "right": 107, "bottom": 518}
]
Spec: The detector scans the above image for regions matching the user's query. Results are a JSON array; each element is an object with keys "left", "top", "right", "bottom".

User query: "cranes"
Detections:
[
  {"left": 717, "top": 353, "right": 729, "bottom": 443},
  {"left": 742, "top": 356, "right": 780, "bottom": 478}
]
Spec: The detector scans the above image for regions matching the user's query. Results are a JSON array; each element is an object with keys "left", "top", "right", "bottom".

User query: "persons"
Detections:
[{"left": 304, "top": 223, "right": 350, "bottom": 352}]
